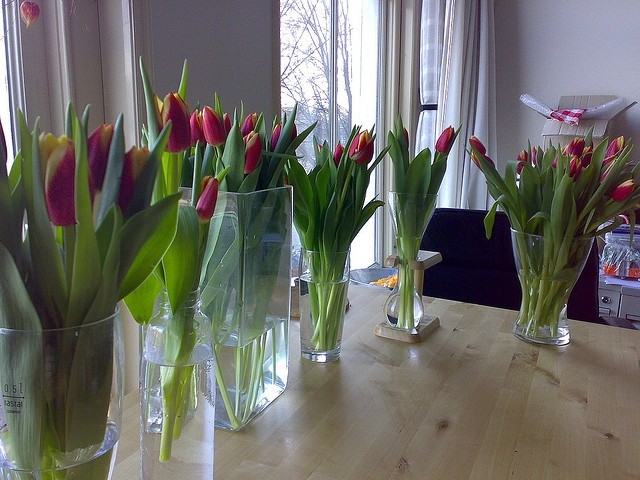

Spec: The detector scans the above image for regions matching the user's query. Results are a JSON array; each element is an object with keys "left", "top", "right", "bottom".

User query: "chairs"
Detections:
[{"left": 421, "top": 208, "right": 638, "bottom": 330}]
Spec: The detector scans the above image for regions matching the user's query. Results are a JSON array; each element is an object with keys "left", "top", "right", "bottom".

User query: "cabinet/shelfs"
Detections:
[{"left": 598, "top": 281, "right": 640, "bottom": 323}]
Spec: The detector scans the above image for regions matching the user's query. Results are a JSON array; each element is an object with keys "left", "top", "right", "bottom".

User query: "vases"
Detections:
[
  {"left": 180, "top": 185, "right": 296, "bottom": 430},
  {"left": 0, "top": 309, "right": 122, "bottom": 479},
  {"left": 383, "top": 191, "right": 438, "bottom": 329},
  {"left": 298, "top": 249, "right": 350, "bottom": 363},
  {"left": 510, "top": 228, "right": 594, "bottom": 345},
  {"left": 136, "top": 291, "right": 218, "bottom": 480}
]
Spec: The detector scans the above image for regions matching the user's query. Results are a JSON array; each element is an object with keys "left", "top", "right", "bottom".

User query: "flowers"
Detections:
[
  {"left": 383, "top": 115, "right": 463, "bottom": 326},
  {"left": 0, "top": 92, "right": 179, "bottom": 480},
  {"left": 123, "top": 54, "right": 226, "bottom": 464},
  {"left": 466, "top": 134, "right": 640, "bottom": 336},
  {"left": 286, "top": 124, "right": 392, "bottom": 348},
  {"left": 181, "top": 94, "right": 316, "bottom": 424}
]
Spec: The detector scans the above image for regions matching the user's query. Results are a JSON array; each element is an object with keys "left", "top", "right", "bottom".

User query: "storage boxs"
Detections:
[{"left": 523, "top": 93, "right": 638, "bottom": 155}]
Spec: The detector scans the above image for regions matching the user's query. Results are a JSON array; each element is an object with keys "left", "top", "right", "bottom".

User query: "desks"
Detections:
[{"left": 97, "top": 284, "right": 640, "bottom": 479}]
